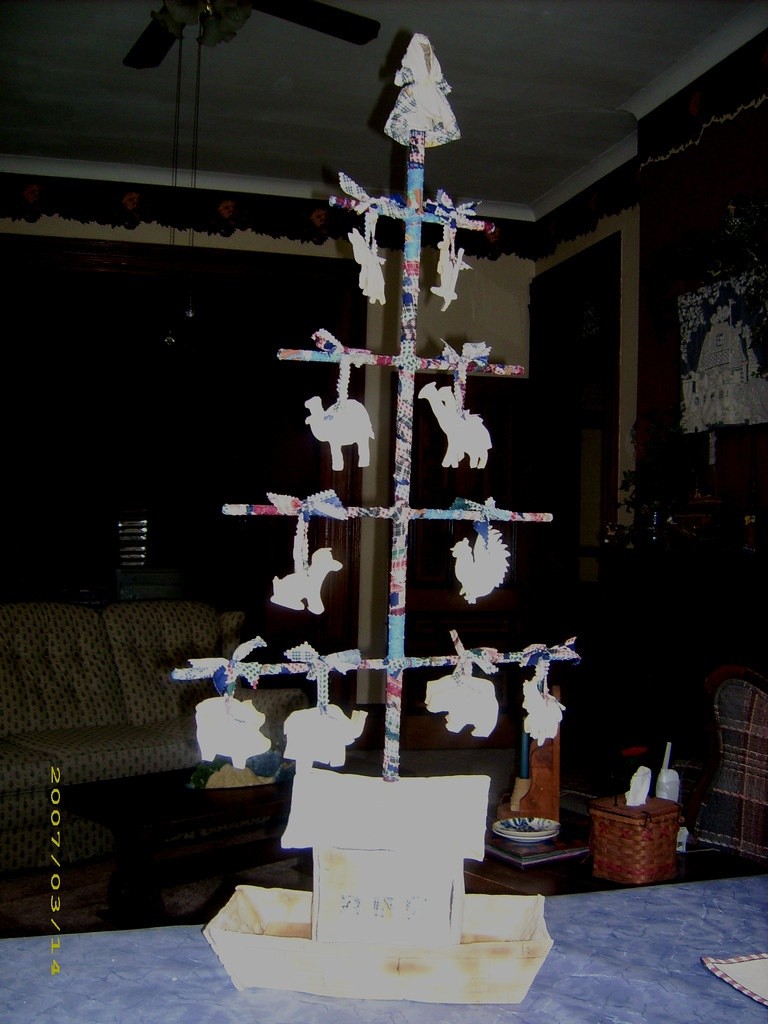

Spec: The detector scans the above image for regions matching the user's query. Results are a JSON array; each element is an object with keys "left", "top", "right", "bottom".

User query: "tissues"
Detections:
[{"left": 587, "top": 765, "right": 683, "bottom": 885}]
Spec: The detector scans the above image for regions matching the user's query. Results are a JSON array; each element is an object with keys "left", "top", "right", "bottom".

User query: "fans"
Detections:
[{"left": 122, "top": 0, "right": 382, "bottom": 70}]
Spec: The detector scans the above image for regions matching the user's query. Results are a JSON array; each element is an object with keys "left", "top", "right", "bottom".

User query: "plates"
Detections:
[{"left": 492, "top": 817, "right": 561, "bottom": 843}]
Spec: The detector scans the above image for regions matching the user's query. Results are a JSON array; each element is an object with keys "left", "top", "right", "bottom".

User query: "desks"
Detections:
[
  {"left": 464, "top": 805, "right": 768, "bottom": 897},
  {"left": 44, "top": 756, "right": 417, "bottom": 930},
  {"left": 0, "top": 876, "right": 768, "bottom": 1024}
]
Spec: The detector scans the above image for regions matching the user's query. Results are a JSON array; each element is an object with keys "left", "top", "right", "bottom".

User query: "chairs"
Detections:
[{"left": 684, "top": 663, "right": 767, "bottom": 865}]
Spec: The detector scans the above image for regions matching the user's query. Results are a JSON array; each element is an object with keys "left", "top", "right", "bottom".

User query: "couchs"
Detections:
[{"left": 0, "top": 598, "right": 311, "bottom": 875}]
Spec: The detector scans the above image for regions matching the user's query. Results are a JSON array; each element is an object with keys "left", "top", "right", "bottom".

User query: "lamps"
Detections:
[{"left": 151, "top": 0, "right": 252, "bottom": 48}]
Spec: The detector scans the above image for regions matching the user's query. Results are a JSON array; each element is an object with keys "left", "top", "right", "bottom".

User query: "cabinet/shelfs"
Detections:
[{"left": 382, "top": 369, "right": 527, "bottom": 750}]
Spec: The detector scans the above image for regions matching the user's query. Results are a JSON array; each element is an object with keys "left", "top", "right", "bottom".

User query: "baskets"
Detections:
[{"left": 588, "top": 794, "right": 680, "bottom": 884}]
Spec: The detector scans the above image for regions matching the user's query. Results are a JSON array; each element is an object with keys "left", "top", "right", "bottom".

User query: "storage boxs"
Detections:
[{"left": 202, "top": 884, "right": 554, "bottom": 1004}]
